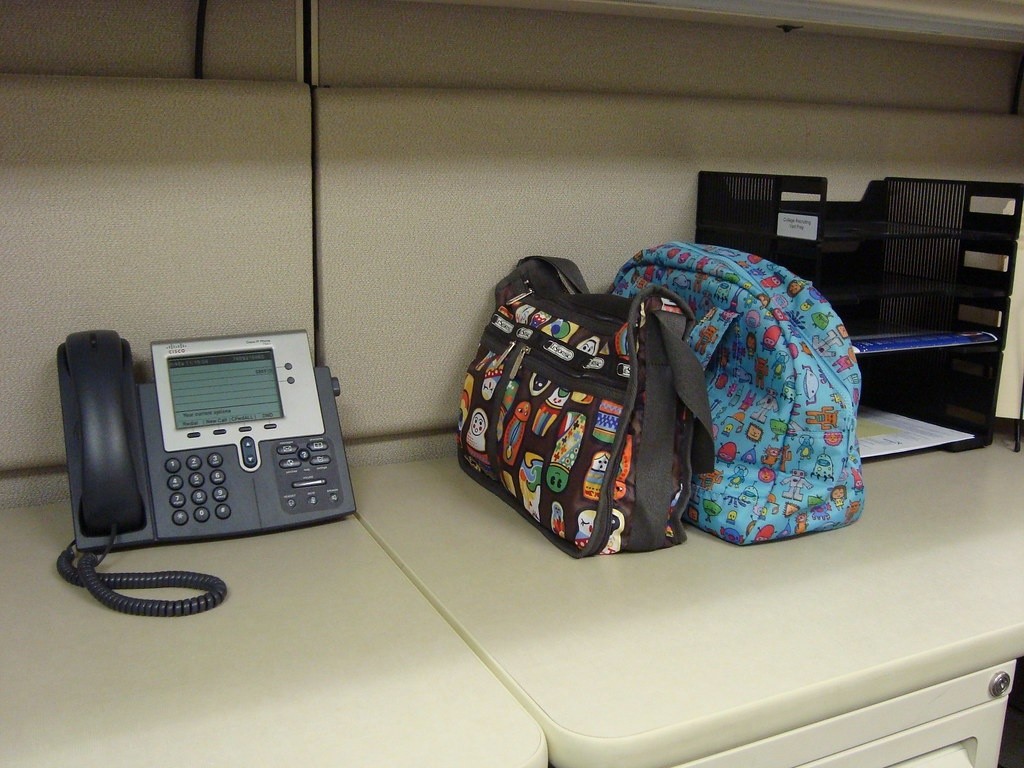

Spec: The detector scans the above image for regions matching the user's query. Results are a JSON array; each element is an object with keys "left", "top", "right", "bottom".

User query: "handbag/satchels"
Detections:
[
  {"left": 610, "top": 239, "right": 866, "bottom": 546},
  {"left": 455, "top": 255, "right": 716, "bottom": 559}
]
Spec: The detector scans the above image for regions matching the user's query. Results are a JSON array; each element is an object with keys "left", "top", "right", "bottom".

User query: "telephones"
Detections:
[{"left": 56, "top": 329, "right": 357, "bottom": 552}]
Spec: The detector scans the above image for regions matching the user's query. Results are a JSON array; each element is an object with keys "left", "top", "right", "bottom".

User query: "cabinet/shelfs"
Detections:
[{"left": 0, "top": 0, "right": 1024, "bottom": 768}]
